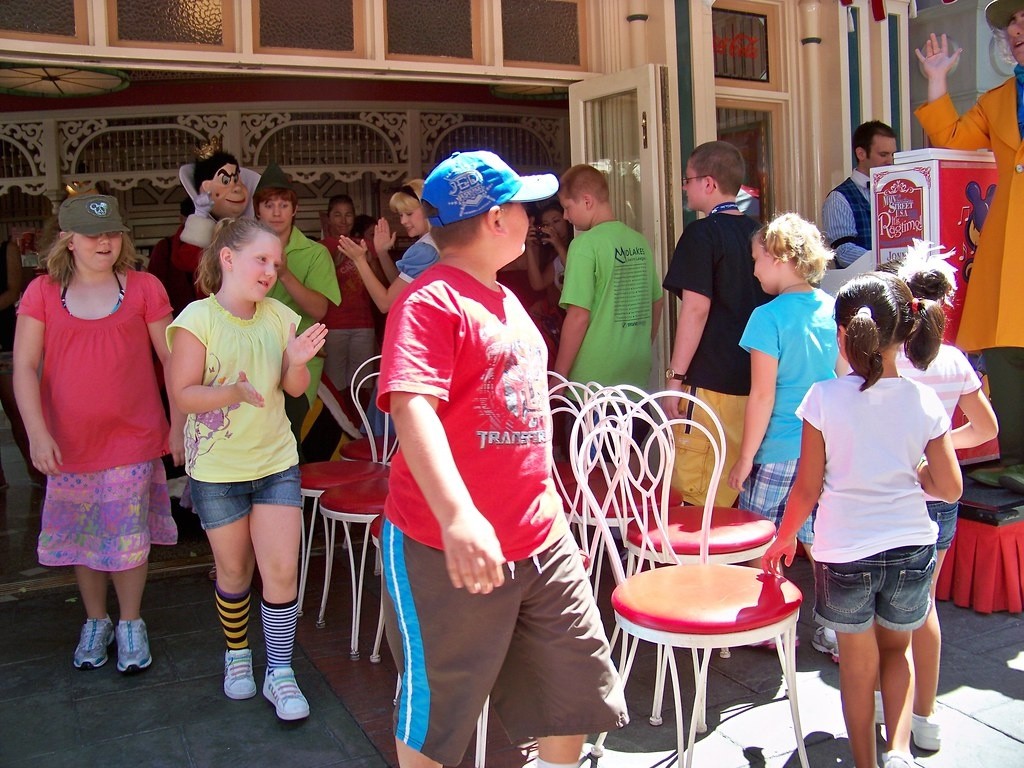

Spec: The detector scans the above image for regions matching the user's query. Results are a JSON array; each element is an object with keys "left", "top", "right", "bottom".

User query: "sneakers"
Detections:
[
  {"left": 911, "top": 710, "right": 941, "bottom": 751},
  {"left": 263, "top": 666, "right": 310, "bottom": 720},
  {"left": 116, "top": 620, "right": 152, "bottom": 672},
  {"left": 811, "top": 626, "right": 840, "bottom": 661},
  {"left": 750, "top": 636, "right": 799, "bottom": 650},
  {"left": 73, "top": 614, "right": 114, "bottom": 668},
  {"left": 224, "top": 647, "right": 256, "bottom": 699},
  {"left": 884, "top": 749, "right": 914, "bottom": 768}
]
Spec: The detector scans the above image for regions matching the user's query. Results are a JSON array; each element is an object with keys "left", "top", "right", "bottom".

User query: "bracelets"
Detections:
[{"left": 916, "top": 456, "right": 928, "bottom": 472}]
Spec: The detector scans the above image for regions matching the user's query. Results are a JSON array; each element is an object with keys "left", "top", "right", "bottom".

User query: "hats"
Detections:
[
  {"left": 983, "top": 0, "right": 1024, "bottom": 29},
  {"left": 421, "top": 150, "right": 560, "bottom": 227},
  {"left": 60, "top": 194, "right": 130, "bottom": 237},
  {"left": 255, "top": 159, "right": 292, "bottom": 188}
]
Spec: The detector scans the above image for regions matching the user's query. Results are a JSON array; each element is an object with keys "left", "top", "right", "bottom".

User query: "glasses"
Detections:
[{"left": 681, "top": 174, "right": 717, "bottom": 189}]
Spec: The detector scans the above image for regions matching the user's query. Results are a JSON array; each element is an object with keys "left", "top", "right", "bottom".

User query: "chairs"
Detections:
[{"left": 298, "top": 355, "right": 819, "bottom": 768}]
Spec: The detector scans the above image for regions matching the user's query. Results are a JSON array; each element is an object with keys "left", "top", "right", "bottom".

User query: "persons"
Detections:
[
  {"left": 913, "top": 0, "right": 1024, "bottom": 495},
  {"left": 374, "top": 150, "right": 633, "bottom": 768},
  {"left": 660, "top": 140, "right": 780, "bottom": 510},
  {"left": 761, "top": 269, "right": 964, "bottom": 768},
  {"left": 11, "top": 192, "right": 187, "bottom": 678},
  {"left": 305, "top": 179, "right": 575, "bottom": 437},
  {"left": 144, "top": 196, "right": 208, "bottom": 320},
  {"left": 18, "top": 227, "right": 45, "bottom": 303},
  {"left": 0, "top": 239, "right": 46, "bottom": 491},
  {"left": 169, "top": 136, "right": 263, "bottom": 300},
  {"left": 821, "top": 120, "right": 899, "bottom": 270},
  {"left": 208, "top": 159, "right": 343, "bottom": 582},
  {"left": 875, "top": 238, "right": 1000, "bottom": 751},
  {"left": 550, "top": 161, "right": 665, "bottom": 464},
  {"left": 164, "top": 214, "right": 330, "bottom": 722},
  {"left": 727, "top": 212, "right": 847, "bottom": 665}
]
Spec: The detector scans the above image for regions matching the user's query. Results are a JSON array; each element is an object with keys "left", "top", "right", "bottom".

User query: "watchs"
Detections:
[{"left": 664, "top": 368, "right": 688, "bottom": 381}]
